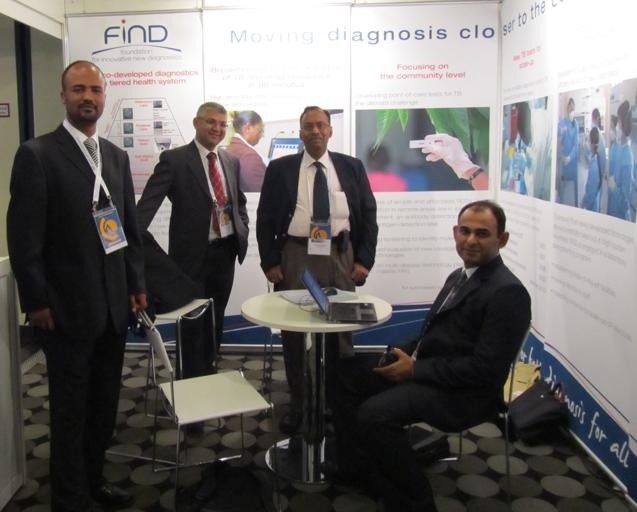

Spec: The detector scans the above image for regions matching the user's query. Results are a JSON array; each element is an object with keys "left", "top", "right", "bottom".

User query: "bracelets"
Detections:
[{"left": 468, "top": 168, "right": 483, "bottom": 182}]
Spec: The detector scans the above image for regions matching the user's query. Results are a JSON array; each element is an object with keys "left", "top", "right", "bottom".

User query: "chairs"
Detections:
[
  {"left": 399, "top": 313, "right": 533, "bottom": 512},
  {"left": 132, "top": 295, "right": 286, "bottom": 512},
  {"left": 121, "top": 216, "right": 224, "bottom": 426}
]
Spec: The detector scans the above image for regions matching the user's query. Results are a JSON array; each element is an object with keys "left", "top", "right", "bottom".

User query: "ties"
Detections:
[
  {"left": 84, "top": 136, "right": 99, "bottom": 166},
  {"left": 311, "top": 161, "right": 331, "bottom": 220},
  {"left": 206, "top": 152, "right": 225, "bottom": 236},
  {"left": 422, "top": 269, "right": 467, "bottom": 339}
]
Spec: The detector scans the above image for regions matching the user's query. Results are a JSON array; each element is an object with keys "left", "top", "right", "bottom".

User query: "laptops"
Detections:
[
  {"left": 301, "top": 268, "right": 381, "bottom": 324},
  {"left": 268, "top": 138, "right": 303, "bottom": 163}
]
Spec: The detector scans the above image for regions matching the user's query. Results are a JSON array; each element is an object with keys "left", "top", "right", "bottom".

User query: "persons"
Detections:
[
  {"left": 133, "top": 102, "right": 249, "bottom": 433},
  {"left": 333, "top": 201, "right": 532, "bottom": 512},
  {"left": 562, "top": 98, "right": 636, "bottom": 221},
  {"left": 418, "top": 131, "right": 489, "bottom": 191},
  {"left": 257, "top": 107, "right": 382, "bottom": 428},
  {"left": 6, "top": 58, "right": 144, "bottom": 512},
  {"left": 501, "top": 105, "right": 552, "bottom": 200},
  {"left": 228, "top": 107, "right": 272, "bottom": 192}
]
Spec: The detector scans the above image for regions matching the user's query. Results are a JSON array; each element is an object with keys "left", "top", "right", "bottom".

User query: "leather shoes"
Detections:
[
  {"left": 278, "top": 408, "right": 307, "bottom": 434},
  {"left": 88, "top": 477, "right": 134, "bottom": 510},
  {"left": 51, "top": 490, "right": 100, "bottom": 511}
]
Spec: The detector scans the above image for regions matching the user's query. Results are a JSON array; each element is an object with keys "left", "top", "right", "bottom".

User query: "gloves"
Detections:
[{"left": 421, "top": 133, "right": 479, "bottom": 178}]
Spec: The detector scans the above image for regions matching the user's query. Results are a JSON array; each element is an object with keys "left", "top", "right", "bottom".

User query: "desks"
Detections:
[{"left": 239, "top": 286, "right": 396, "bottom": 496}]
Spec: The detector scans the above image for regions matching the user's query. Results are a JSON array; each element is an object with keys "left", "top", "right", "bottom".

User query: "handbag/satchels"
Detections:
[{"left": 505, "top": 380, "right": 571, "bottom": 445}]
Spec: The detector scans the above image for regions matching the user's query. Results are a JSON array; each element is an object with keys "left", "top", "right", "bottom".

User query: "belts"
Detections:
[{"left": 294, "top": 235, "right": 340, "bottom": 248}]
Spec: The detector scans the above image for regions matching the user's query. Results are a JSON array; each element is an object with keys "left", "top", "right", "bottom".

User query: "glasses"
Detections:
[
  {"left": 197, "top": 116, "right": 228, "bottom": 128},
  {"left": 303, "top": 122, "right": 328, "bottom": 130}
]
